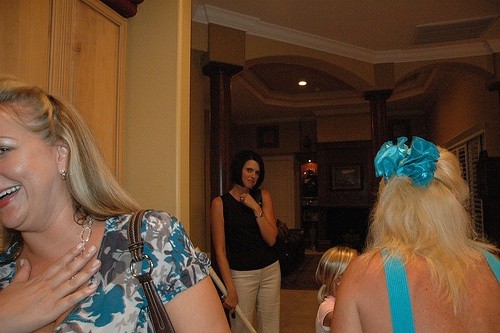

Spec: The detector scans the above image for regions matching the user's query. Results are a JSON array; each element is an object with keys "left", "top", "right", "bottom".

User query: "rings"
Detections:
[{"left": 241, "top": 198, "right": 243, "bottom": 199}]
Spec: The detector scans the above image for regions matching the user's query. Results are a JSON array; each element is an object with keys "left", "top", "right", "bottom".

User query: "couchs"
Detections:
[{"left": 274, "top": 219, "right": 305, "bottom": 277}]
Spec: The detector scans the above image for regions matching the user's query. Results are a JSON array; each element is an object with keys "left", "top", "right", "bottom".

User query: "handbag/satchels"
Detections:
[{"left": 276, "top": 222, "right": 306, "bottom": 275}]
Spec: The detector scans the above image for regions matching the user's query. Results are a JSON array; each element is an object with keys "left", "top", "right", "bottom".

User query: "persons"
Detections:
[
  {"left": 211, "top": 150, "right": 281, "bottom": 333},
  {"left": 315, "top": 247, "right": 359, "bottom": 333},
  {"left": 330, "top": 134, "right": 500, "bottom": 333},
  {"left": 0, "top": 84, "right": 231, "bottom": 333}
]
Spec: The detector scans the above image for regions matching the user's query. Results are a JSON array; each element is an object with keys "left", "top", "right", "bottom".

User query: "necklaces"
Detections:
[{"left": 80, "top": 216, "right": 94, "bottom": 254}]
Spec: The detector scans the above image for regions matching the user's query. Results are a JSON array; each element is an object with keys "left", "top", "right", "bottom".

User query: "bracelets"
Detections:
[{"left": 255, "top": 211, "right": 264, "bottom": 218}]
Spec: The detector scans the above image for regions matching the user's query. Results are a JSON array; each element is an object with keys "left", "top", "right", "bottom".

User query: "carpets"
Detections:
[{"left": 281, "top": 253, "right": 324, "bottom": 290}]
